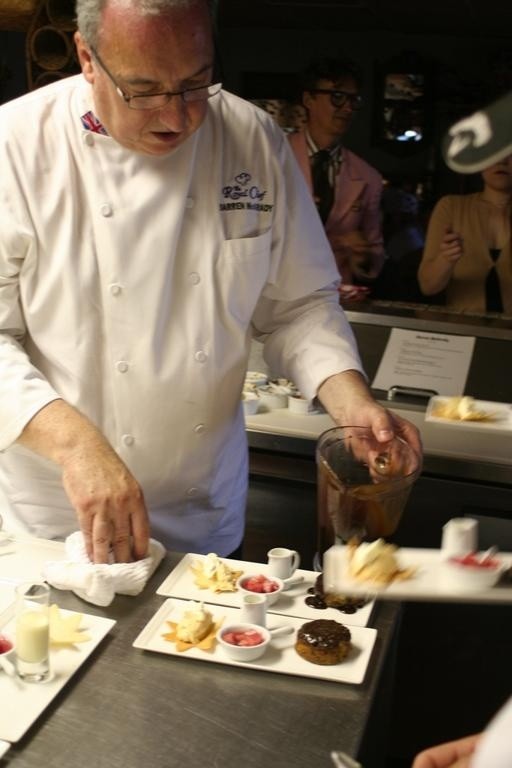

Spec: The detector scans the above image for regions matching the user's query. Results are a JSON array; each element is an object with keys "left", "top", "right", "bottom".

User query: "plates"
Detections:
[
  {"left": 422, "top": 390, "right": 512, "bottom": 437},
  {"left": 154, "top": 551, "right": 379, "bottom": 628},
  {"left": 130, "top": 598, "right": 380, "bottom": 686},
  {"left": 0, "top": 597, "right": 118, "bottom": 744},
  {"left": 319, "top": 538, "right": 509, "bottom": 603}
]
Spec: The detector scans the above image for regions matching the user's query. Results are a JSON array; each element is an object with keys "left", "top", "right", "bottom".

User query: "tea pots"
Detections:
[{"left": 312, "top": 426, "right": 423, "bottom": 572}]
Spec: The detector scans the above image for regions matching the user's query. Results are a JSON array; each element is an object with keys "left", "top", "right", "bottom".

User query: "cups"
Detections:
[
  {"left": 241, "top": 594, "right": 266, "bottom": 628},
  {"left": 267, "top": 546, "right": 303, "bottom": 580},
  {"left": 442, "top": 515, "right": 478, "bottom": 565},
  {"left": 13, "top": 579, "right": 53, "bottom": 685}
]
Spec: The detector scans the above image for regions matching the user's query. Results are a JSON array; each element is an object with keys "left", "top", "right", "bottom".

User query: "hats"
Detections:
[{"left": 443, "top": 97, "right": 512, "bottom": 174}]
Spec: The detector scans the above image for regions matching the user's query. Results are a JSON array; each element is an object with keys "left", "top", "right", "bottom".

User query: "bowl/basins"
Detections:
[
  {"left": 441, "top": 549, "right": 505, "bottom": 591},
  {"left": 239, "top": 371, "right": 314, "bottom": 415},
  {"left": 236, "top": 573, "right": 282, "bottom": 608},
  {"left": 217, "top": 622, "right": 271, "bottom": 661}
]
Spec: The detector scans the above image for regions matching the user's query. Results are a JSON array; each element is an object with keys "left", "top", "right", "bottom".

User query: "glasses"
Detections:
[
  {"left": 91, "top": 49, "right": 223, "bottom": 113},
  {"left": 312, "top": 88, "right": 365, "bottom": 111}
]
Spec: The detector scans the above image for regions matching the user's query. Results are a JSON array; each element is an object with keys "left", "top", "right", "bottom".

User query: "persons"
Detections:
[
  {"left": 362, "top": 98, "right": 442, "bottom": 302},
  {"left": 412, "top": 695, "right": 511, "bottom": 768},
  {"left": 0, "top": 1, "right": 423, "bottom": 569},
  {"left": 416, "top": 150, "right": 512, "bottom": 318},
  {"left": 284, "top": 61, "right": 388, "bottom": 304}
]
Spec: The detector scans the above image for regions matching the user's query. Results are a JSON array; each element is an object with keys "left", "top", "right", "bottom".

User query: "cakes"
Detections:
[{"left": 295, "top": 620, "right": 352, "bottom": 666}]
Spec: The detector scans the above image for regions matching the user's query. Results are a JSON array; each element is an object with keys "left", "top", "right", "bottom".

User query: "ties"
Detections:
[{"left": 311, "top": 149, "right": 331, "bottom": 209}]
